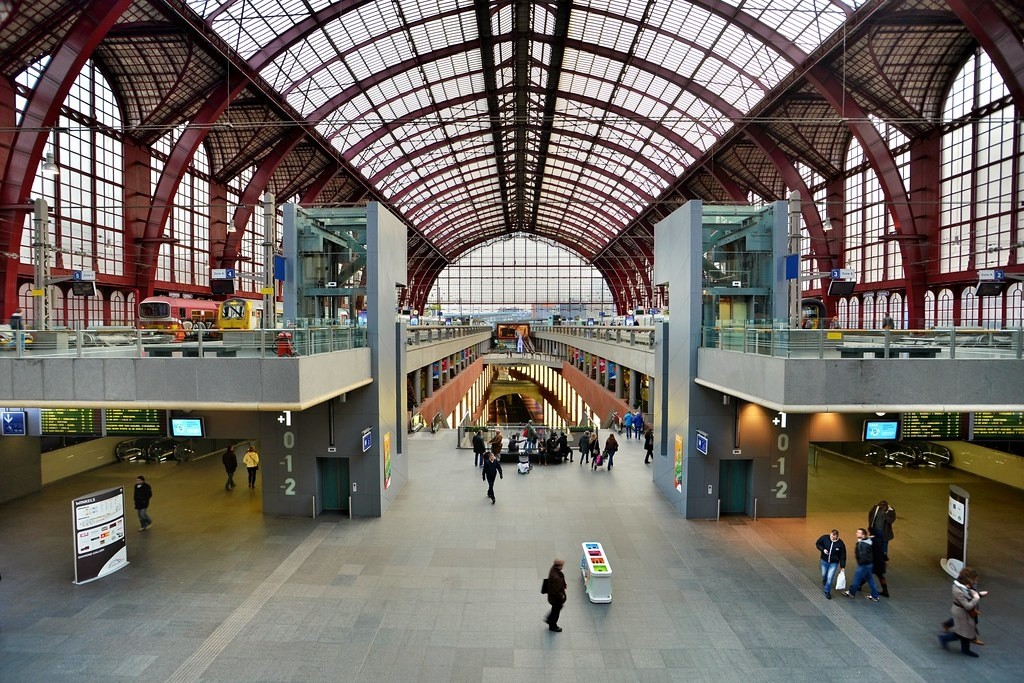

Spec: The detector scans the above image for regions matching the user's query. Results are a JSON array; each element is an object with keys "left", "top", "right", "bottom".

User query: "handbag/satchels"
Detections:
[
  {"left": 256, "top": 464, "right": 258, "bottom": 470},
  {"left": 593, "top": 449, "right": 598, "bottom": 454},
  {"left": 968, "top": 609, "right": 977, "bottom": 617},
  {"left": 835, "top": 569, "right": 846, "bottom": 590},
  {"left": 603, "top": 451, "right": 608, "bottom": 458},
  {"left": 541, "top": 578, "right": 549, "bottom": 594},
  {"left": 596, "top": 455, "right": 603, "bottom": 466}
]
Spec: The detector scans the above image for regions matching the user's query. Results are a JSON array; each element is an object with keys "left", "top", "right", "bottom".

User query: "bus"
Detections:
[
  {"left": 137, "top": 293, "right": 229, "bottom": 342},
  {"left": 216, "top": 298, "right": 285, "bottom": 334}
]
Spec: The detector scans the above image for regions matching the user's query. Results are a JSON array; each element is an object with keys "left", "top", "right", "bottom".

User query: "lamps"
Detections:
[
  {"left": 41, "top": 132, "right": 59, "bottom": 174},
  {"left": 104, "top": 239, "right": 112, "bottom": 249},
  {"left": 953, "top": 236, "right": 960, "bottom": 247},
  {"left": 227, "top": 219, "right": 236, "bottom": 232},
  {"left": 823, "top": 216, "right": 833, "bottom": 230}
]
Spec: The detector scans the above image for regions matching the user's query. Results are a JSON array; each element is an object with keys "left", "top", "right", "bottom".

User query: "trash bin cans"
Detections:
[{"left": 581, "top": 541, "right": 613, "bottom": 604}]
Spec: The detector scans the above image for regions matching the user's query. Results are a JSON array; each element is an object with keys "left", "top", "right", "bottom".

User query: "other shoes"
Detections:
[
  {"left": 841, "top": 591, "right": 854, "bottom": 598},
  {"left": 825, "top": 592, "right": 831, "bottom": 599},
  {"left": 965, "top": 650, "right": 978, "bottom": 657},
  {"left": 493, "top": 499, "right": 495, "bottom": 503},
  {"left": 943, "top": 627, "right": 948, "bottom": 632},
  {"left": 550, "top": 627, "right": 562, "bottom": 632},
  {"left": 864, "top": 595, "right": 878, "bottom": 602},
  {"left": 972, "top": 639, "right": 984, "bottom": 644},
  {"left": 138, "top": 527, "right": 144, "bottom": 531},
  {"left": 145, "top": 522, "right": 152, "bottom": 529},
  {"left": 878, "top": 591, "right": 888, "bottom": 597},
  {"left": 823, "top": 580, "right": 826, "bottom": 585}
]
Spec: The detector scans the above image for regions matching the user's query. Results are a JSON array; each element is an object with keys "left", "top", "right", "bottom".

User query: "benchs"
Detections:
[{"left": 500, "top": 450, "right": 538, "bottom": 463}]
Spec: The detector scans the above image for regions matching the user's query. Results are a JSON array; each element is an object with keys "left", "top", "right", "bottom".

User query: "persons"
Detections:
[
  {"left": 488, "top": 430, "right": 503, "bottom": 457},
  {"left": 829, "top": 316, "right": 841, "bottom": 329},
  {"left": 242, "top": 446, "right": 259, "bottom": 489},
  {"left": 508, "top": 419, "right": 574, "bottom": 466},
  {"left": 482, "top": 453, "right": 503, "bottom": 504},
  {"left": 578, "top": 431, "right": 619, "bottom": 471},
  {"left": 222, "top": 446, "right": 238, "bottom": 491},
  {"left": 843, "top": 528, "right": 880, "bottom": 602},
  {"left": 625, "top": 411, "right": 653, "bottom": 464},
  {"left": 816, "top": 529, "right": 846, "bottom": 599},
  {"left": 134, "top": 475, "right": 152, "bottom": 531},
  {"left": 882, "top": 312, "right": 894, "bottom": 329},
  {"left": 472, "top": 430, "right": 486, "bottom": 468},
  {"left": 544, "top": 559, "right": 567, "bottom": 632},
  {"left": 937, "top": 568, "right": 989, "bottom": 659},
  {"left": 802, "top": 315, "right": 815, "bottom": 329},
  {"left": 856, "top": 501, "right": 897, "bottom": 598},
  {"left": 6, "top": 309, "right": 26, "bottom": 352}
]
